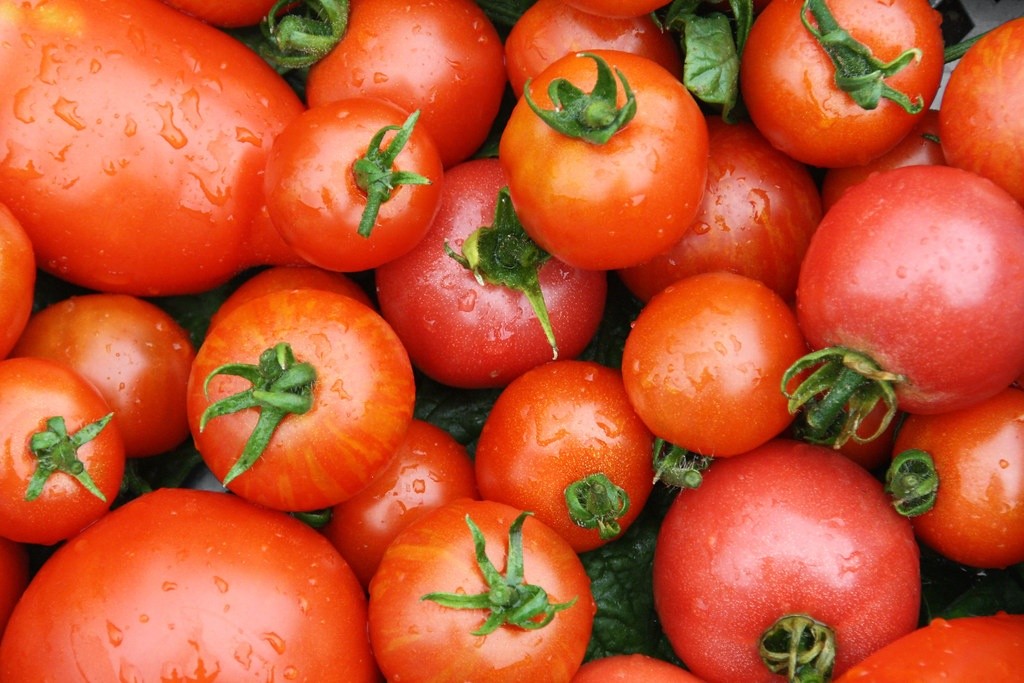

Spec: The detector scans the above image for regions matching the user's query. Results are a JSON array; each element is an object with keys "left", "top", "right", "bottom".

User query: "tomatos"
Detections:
[{"left": 0, "top": 0, "right": 1024, "bottom": 683}]
